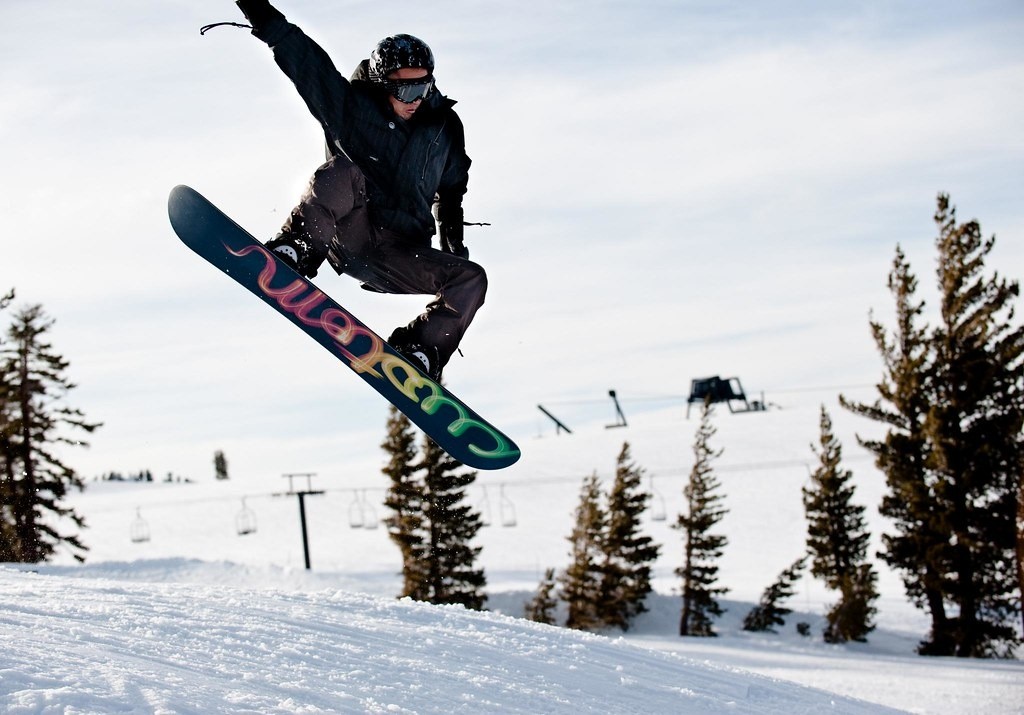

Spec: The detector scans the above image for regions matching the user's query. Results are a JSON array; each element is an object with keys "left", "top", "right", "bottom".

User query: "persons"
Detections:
[{"left": 237, "top": 0, "right": 487, "bottom": 385}]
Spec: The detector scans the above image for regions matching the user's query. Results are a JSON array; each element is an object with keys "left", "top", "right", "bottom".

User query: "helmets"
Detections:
[{"left": 369, "top": 34, "right": 435, "bottom": 86}]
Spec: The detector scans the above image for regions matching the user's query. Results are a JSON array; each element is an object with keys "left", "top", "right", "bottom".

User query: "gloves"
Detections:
[{"left": 236, "top": 0, "right": 273, "bottom": 19}]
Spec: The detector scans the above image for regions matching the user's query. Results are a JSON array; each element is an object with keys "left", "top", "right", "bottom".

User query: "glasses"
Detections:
[{"left": 392, "top": 77, "right": 434, "bottom": 104}]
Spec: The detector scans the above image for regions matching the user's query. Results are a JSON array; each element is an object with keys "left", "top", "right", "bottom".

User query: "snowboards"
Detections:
[{"left": 167, "top": 183, "right": 522, "bottom": 471}]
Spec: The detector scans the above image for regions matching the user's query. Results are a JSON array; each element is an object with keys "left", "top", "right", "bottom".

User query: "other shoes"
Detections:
[
  {"left": 265, "top": 230, "right": 324, "bottom": 279},
  {"left": 389, "top": 326, "right": 442, "bottom": 383}
]
